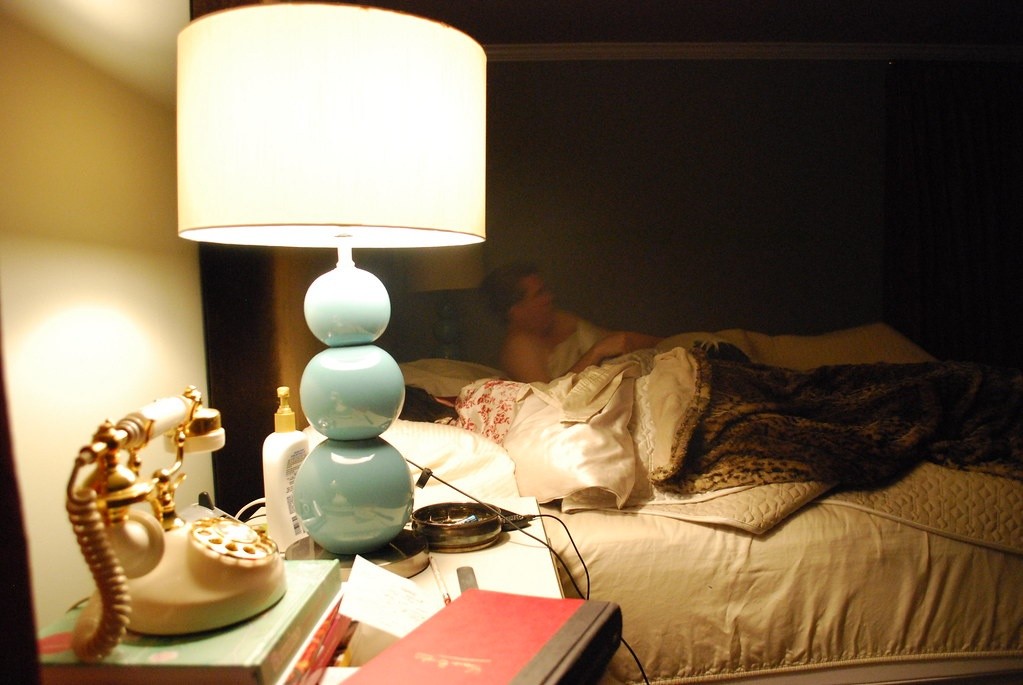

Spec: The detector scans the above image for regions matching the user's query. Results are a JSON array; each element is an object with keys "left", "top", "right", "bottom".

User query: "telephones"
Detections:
[{"left": 71, "top": 388, "right": 289, "bottom": 663}]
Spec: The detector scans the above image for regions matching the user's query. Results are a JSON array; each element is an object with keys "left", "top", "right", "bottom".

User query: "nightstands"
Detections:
[{"left": 281, "top": 494, "right": 564, "bottom": 685}]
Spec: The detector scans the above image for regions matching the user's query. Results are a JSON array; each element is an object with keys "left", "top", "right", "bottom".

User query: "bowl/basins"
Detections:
[{"left": 411, "top": 502, "right": 501, "bottom": 552}]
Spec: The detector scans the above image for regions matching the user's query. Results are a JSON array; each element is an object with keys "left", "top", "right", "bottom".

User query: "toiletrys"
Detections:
[{"left": 263, "top": 386, "right": 309, "bottom": 553}]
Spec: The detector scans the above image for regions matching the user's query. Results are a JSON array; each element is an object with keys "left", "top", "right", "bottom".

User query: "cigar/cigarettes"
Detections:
[{"left": 457, "top": 516, "right": 477, "bottom": 524}]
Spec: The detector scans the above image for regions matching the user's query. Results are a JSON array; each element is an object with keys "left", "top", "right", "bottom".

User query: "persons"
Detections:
[
  {"left": 491, "top": 265, "right": 666, "bottom": 383},
  {"left": 399, "top": 379, "right": 551, "bottom": 445}
]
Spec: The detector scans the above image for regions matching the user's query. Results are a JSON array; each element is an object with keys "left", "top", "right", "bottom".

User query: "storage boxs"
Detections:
[{"left": 344, "top": 588, "right": 623, "bottom": 685}]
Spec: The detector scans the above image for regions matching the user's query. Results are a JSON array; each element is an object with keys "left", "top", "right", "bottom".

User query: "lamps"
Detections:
[{"left": 177, "top": 1, "right": 490, "bottom": 558}]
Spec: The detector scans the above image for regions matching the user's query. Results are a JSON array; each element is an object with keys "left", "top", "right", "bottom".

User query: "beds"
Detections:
[{"left": 394, "top": 321, "right": 1023, "bottom": 685}]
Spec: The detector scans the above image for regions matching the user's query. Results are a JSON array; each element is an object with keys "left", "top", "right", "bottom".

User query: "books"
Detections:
[{"left": 346, "top": 587, "right": 622, "bottom": 685}]
